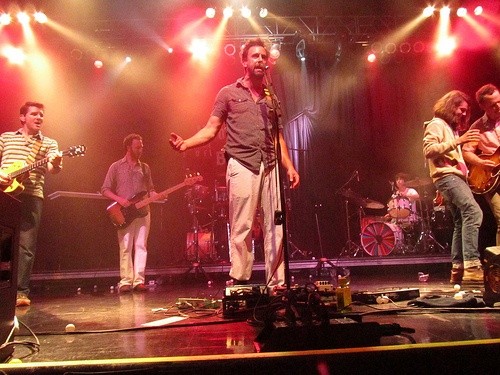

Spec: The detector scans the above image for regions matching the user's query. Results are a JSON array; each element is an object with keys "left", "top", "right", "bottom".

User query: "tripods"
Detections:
[
  {"left": 406, "top": 186, "right": 448, "bottom": 255},
  {"left": 171, "top": 202, "right": 228, "bottom": 265},
  {"left": 337, "top": 198, "right": 363, "bottom": 258}
]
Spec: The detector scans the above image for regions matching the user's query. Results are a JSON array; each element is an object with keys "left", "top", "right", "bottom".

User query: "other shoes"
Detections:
[
  {"left": 134, "top": 284, "right": 148, "bottom": 291},
  {"left": 462, "top": 268, "right": 485, "bottom": 281},
  {"left": 120, "top": 285, "right": 131, "bottom": 292},
  {"left": 16, "top": 293, "right": 31, "bottom": 307},
  {"left": 450, "top": 269, "right": 463, "bottom": 283}
]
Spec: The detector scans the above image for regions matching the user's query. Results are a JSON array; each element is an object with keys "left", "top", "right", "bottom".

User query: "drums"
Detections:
[
  {"left": 387, "top": 197, "right": 411, "bottom": 220},
  {"left": 430, "top": 206, "right": 455, "bottom": 233},
  {"left": 185, "top": 231, "right": 215, "bottom": 262},
  {"left": 397, "top": 211, "right": 420, "bottom": 228},
  {"left": 358, "top": 218, "right": 408, "bottom": 260}
]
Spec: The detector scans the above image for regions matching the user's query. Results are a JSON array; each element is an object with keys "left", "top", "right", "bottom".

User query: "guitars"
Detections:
[
  {"left": 465, "top": 143, "right": 500, "bottom": 196},
  {"left": 105, "top": 171, "right": 205, "bottom": 230},
  {"left": 0, "top": 144, "right": 87, "bottom": 198}
]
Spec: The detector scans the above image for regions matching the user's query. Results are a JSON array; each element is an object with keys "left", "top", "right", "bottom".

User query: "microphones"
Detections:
[
  {"left": 356, "top": 169, "right": 360, "bottom": 182},
  {"left": 258, "top": 62, "right": 269, "bottom": 69}
]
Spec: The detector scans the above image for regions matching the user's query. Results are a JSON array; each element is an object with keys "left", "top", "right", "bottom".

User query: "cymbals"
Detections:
[
  {"left": 403, "top": 177, "right": 432, "bottom": 187},
  {"left": 359, "top": 197, "right": 384, "bottom": 212},
  {"left": 336, "top": 188, "right": 357, "bottom": 199}
]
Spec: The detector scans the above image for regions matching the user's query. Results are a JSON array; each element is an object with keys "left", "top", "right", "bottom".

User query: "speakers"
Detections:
[
  {"left": 484, "top": 246, "right": 500, "bottom": 306},
  {"left": 0, "top": 190, "right": 24, "bottom": 361}
]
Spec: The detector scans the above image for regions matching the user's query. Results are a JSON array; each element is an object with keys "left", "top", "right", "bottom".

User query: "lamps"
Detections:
[
  {"left": 270, "top": 39, "right": 282, "bottom": 58},
  {"left": 254, "top": 5, "right": 268, "bottom": 19},
  {"left": 71, "top": 47, "right": 98, "bottom": 61},
  {"left": 224, "top": 43, "right": 236, "bottom": 54},
  {"left": 336, "top": 39, "right": 351, "bottom": 61},
  {"left": 370, "top": 41, "right": 433, "bottom": 66},
  {"left": 295, "top": 39, "right": 313, "bottom": 61}
]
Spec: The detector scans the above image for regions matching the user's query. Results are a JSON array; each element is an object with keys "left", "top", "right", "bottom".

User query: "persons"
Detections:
[
  {"left": 388, "top": 173, "right": 419, "bottom": 247},
  {"left": 169, "top": 38, "right": 301, "bottom": 285},
  {"left": 0, "top": 102, "right": 62, "bottom": 306},
  {"left": 461, "top": 84, "right": 500, "bottom": 265},
  {"left": 102, "top": 133, "right": 168, "bottom": 294},
  {"left": 422, "top": 91, "right": 483, "bottom": 283}
]
